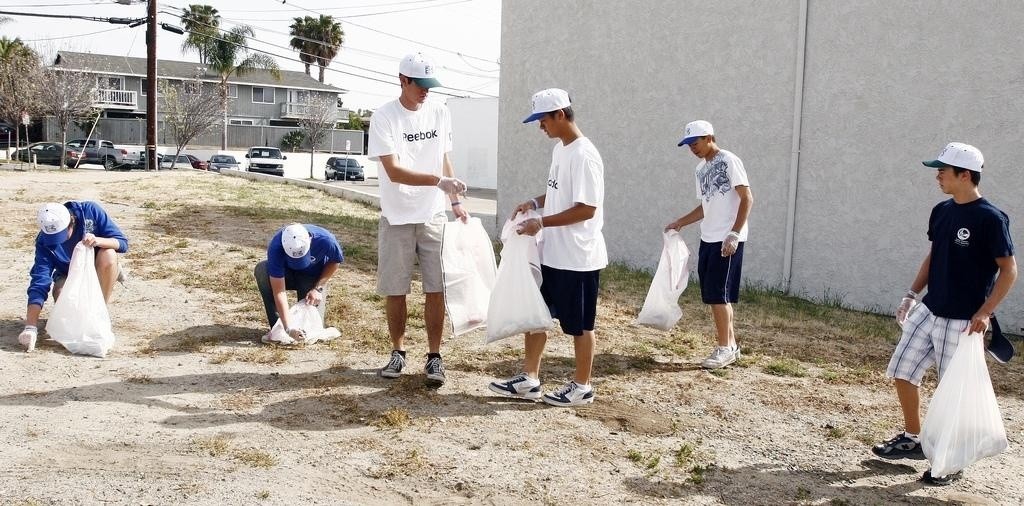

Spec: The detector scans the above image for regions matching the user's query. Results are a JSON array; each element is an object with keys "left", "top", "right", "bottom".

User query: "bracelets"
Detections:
[
  {"left": 906, "top": 287, "right": 917, "bottom": 298},
  {"left": 538, "top": 215, "right": 543, "bottom": 228},
  {"left": 451, "top": 201, "right": 461, "bottom": 207},
  {"left": 531, "top": 198, "right": 539, "bottom": 210}
]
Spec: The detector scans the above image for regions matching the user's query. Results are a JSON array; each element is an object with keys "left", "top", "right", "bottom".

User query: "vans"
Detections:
[{"left": 245, "top": 147, "right": 287, "bottom": 177}]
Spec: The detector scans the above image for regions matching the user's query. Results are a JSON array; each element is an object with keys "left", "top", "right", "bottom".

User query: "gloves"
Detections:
[
  {"left": 720, "top": 229, "right": 740, "bottom": 258},
  {"left": 509, "top": 199, "right": 535, "bottom": 223},
  {"left": 893, "top": 296, "right": 917, "bottom": 331},
  {"left": 302, "top": 288, "right": 323, "bottom": 308},
  {"left": 17, "top": 324, "right": 38, "bottom": 354},
  {"left": 450, "top": 200, "right": 472, "bottom": 225},
  {"left": 435, "top": 175, "right": 469, "bottom": 197},
  {"left": 514, "top": 216, "right": 545, "bottom": 237},
  {"left": 81, "top": 232, "right": 101, "bottom": 248},
  {"left": 284, "top": 325, "right": 308, "bottom": 342}
]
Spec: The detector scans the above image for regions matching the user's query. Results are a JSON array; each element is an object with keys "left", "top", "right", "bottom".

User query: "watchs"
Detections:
[{"left": 314, "top": 284, "right": 323, "bottom": 293}]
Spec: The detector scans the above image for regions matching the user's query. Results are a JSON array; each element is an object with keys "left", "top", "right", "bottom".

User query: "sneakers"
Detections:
[
  {"left": 377, "top": 350, "right": 409, "bottom": 379},
  {"left": 422, "top": 350, "right": 447, "bottom": 385},
  {"left": 540, "top": 379, "right": 594, "bottom": 407},
  {"left": 698, "top": 344, "right": 737, "bottom": 370},
  {"left": 487, "top": 373, "right": 545, "bottom": 401},
  {"left": 920, "top": 464, "right": 967, "bottom": 486},
  {"left": 867, "top": 428, "right": 929, "bottom": 462}
]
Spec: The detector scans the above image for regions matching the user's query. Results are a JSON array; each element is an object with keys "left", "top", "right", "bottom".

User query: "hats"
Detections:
[
  {"left": 281, "top": 222, "right": 312, "bottom": 270},
  {"left": 520, "top": 86, "right": 575, "bottom": 123},
  {"left": 674, "top": 118, "right": 715, "bottom": 146},
  {"left": 920, "top": 140, "right": 988, "bottom": 173},
  {"left": 398, "top": 51, "right": 444, "bottom": 90},
  {"left": 36, "top": 201, "right": 71, "bottom": 247},
  {"left": 982, "top": 313, "right": 1016, "bottom": 365}
]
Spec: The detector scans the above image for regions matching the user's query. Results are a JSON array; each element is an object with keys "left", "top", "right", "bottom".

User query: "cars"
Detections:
[
  {"left": 207, "top": 154, "right": 239, "bottom": 171},
  {"left": 11, "top": 141, "right": 88, "bottom": 169},
  {"left": 131, "top": 151, "right": 208, "bottom": 172},
  {"left": 324, "top": 156, "right": 365, "bottom": 181}
]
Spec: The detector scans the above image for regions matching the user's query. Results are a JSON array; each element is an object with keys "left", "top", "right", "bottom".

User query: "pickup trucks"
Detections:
[{"left": 66, "top": 138, "right": 141, "bottom": 170}]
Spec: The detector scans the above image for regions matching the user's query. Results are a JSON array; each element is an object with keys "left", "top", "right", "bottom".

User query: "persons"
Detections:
[
  {"left": 872, "top": 142, "right": 1018, "bottom": 488},
  {"left": 254, "top": 223, "right": 343, "bottom": 343},
  {"left": 365, "top": 52, "right": 470, "bottom": 385},
  {"left": 664, "top": 118, "right": 753, "bottom": 368},
  {"left": 483, "top": 86, "right": 608, "bottom": 408},
  {"left": 17, "top": 200, "right": 129, "bottom": 353}
]
